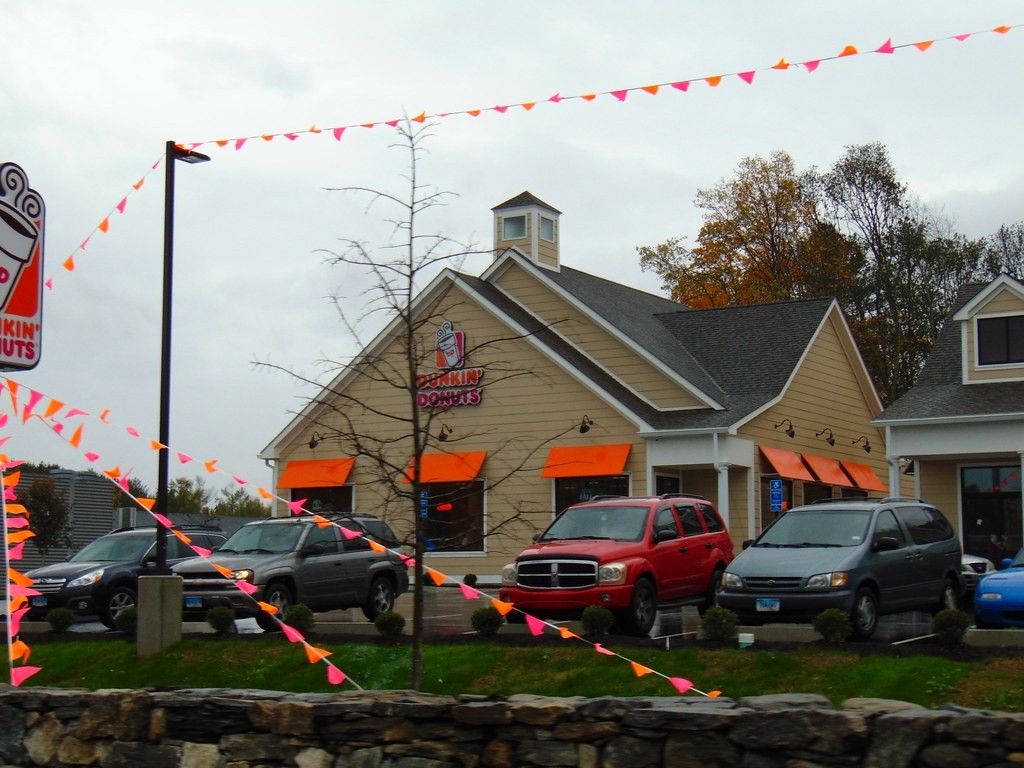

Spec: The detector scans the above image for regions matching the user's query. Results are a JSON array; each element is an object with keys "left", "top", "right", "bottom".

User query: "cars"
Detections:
[{"left": 973, "top": 548, "right": 1024, "bottom": 628}]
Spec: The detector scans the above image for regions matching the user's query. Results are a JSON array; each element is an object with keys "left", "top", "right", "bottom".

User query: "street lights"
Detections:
[{"left": 153, "top": 141, "right": 214, "bottom": 575}]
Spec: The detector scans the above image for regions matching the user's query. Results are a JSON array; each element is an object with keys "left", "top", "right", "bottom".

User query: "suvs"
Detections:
[
  {"left": 15, "top": 522, "right": 236, "bottom": 631},
  {"left": 717, "top": 495, "right": 966, "bottom": 643},
  {"left": 498, "top": 492, "right": 735, "bottom": 637},
  {"left": 169, "top": 510, "right": 409, "bottom": 631}
]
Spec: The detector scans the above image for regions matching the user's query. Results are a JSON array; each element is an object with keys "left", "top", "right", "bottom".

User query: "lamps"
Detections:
[
  {"left": 853, "top": 436, "right": 872, "bottom": 453},
  {"left": 817, "top": 429, "right": 834, "bottom": 446},
  {"left": 310, "top": 432, "right": 323, "bottom": 448},
  {"left": 581, "top": 414, "right": 592, "bottom": 434},
  {"left": 776, "top": 419, "right": 794, "bottom": 439},
  {"left": 439, "top": 424, "right": 452, "bottom": 440}
]
